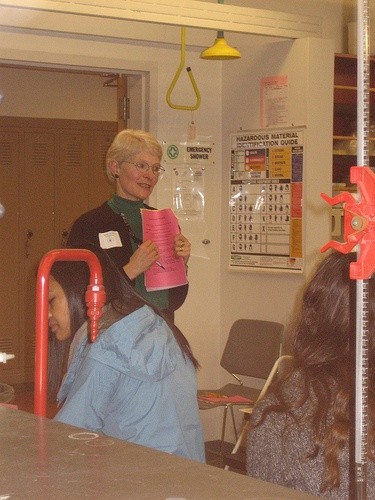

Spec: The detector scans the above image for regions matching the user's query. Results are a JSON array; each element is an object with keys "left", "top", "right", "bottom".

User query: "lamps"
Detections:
[{"left": 200, "top": 0, "right": 240, "bottom": 60}]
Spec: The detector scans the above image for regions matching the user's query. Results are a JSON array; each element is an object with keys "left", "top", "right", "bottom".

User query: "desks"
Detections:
[{"left": 0, "top": 403, "right": 325, "bottom": 500}]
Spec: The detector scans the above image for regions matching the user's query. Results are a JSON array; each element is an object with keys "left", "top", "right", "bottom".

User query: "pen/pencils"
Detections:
[{"left": 137, "top": 245, "right": 166, "bottom": 270}]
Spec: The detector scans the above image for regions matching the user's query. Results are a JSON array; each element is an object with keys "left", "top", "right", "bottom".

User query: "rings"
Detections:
[
  {"left": 181, "top": 242, "right": 183, "bottom": 246},
  {"left": 181, "top": 247, "right": 184, "bottom": 251},
  {"left": 152, "top": 251, "right": 157, "bottom": 255}
]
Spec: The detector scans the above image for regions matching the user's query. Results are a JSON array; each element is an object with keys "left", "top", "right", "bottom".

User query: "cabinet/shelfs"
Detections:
[{"left": 334, "top": 53, "right": 375, "bottom": 190}]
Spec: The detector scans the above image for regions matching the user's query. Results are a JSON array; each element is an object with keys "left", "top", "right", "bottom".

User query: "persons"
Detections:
[
  {"left": 46, "top": 240, "right": 207, "bottom": 464},
  {"left": 246, "top": 250, "right": 375, "bottom": 500},
  {"left": 63, "top": 128, "right": 191, "bottom": 322}
]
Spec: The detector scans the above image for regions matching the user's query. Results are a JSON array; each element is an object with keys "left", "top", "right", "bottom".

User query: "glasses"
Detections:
[{"left": 118, "top": 159, "right": 165, "bottom": 176}]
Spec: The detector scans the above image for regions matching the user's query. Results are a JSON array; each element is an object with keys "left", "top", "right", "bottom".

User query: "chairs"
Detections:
[
  {"left": 223, "top": 355, "right": 297, "bottom": 475},
  {"left": 197, "top": 319, "right": 288, "bottom": 467}
]
